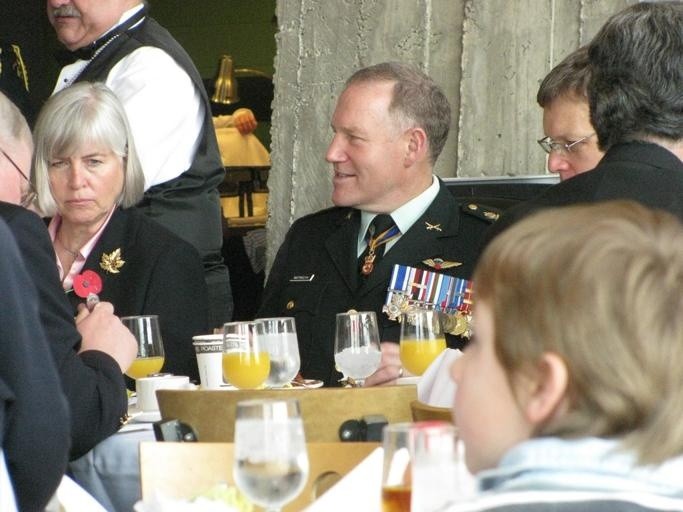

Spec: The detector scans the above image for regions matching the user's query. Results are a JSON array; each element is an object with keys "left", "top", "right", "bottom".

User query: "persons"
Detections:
[
  {"left": 477, "top": 0, "right": 683, "bottom": 257},
  {"left": 0, "top": 218, "right": 71, "bottom": 511},
  {"left": 0, "top": 92, "right": 140, "bottom": 466},
  {"left": 211, "top": 61, "right": 506, "bottom": 392},
  {"left": 209, "top": 107, "right": 259, "bottom": 136},
  {"left": 533, "top": 44, "right": 606, "bottom": 184},
  {"left": 42, "top": 1, "right": 234, "bottom": 337},
  {"left": 25, "top": 79, "right": 204, "bottom": 383},
  {"left": 429, "top": 198, "right": 683, "bottom": 512}
]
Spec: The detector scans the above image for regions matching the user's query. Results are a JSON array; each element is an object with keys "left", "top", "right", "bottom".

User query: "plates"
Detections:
[
  {"left": 282, "top": 377, "right": 324, "bottom": 389},
  {"left": 134, "top": 412, "right": 161, "bottom": 423}
]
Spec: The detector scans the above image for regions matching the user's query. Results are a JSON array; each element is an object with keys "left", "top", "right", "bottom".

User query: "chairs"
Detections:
[
  {"left": 139, "top": 439, "right": 381, "bottom": 512},
  {"left": 410, "top": 399, "right": 453, "bottom": 422},
  {"left": 155, "top": 383, "right": 418, "bottom": 441}
]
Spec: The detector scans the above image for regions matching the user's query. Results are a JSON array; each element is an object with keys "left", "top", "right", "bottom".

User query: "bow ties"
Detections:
[{"left": 58, "top": 41, "right": 97, "bottom": 66}]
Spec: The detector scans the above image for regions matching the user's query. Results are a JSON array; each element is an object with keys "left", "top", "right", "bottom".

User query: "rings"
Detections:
[{"left": 397, "top": 365, "right": 404, "bottom": 378}]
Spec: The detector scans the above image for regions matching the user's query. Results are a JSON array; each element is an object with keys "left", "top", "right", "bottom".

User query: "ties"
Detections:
[{"left": 357, "top": 215, "right": 402, "bottom": 281}]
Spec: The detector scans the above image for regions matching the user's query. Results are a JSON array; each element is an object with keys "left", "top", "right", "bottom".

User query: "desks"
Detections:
[{"left": 214, "top": 127, "right": 272, "bottom": 217}]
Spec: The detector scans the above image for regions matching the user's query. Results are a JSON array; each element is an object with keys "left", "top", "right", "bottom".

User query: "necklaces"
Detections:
[{"left": 55, "top": 230, "right": 76, "bottom": 255}]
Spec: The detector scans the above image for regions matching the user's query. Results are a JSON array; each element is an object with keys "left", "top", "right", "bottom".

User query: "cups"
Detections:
[
  {"left": 137, "top": 377, "right": 194, "bottom": 414},
  {"left": 121, "top": 315, "right": 164, "bottom": 376},
  {"left": 334, "top": 312, "right": 382, "bottom": 387},
  {"left": 233, "top": 401, "right": 309, "bottom": 512},
  {"left": 193, "top": 334, "right": 224, "bottom": 342},
  {"left": 222, "top": 322, "right": 269, "bottom": 390},
  {"left": 195, "top": 343, "right": 223, "bottom": 386},
  {"left": 383, "top": 422, "right": 456, "bottom": 512},
  {"left": 401, "top": 310, "right": 446, "bottom": 376},
  {"left": 253, "top": 318, "right": 301, "bottom": 387}
]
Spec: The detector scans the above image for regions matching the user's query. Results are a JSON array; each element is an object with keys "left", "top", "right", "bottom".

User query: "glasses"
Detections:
[
  {"left": 0, "top": 149, "right": 39, "bottom": 209},
  {"left": 538, "top": 134, "right": 598, "bottom": 157}
]
